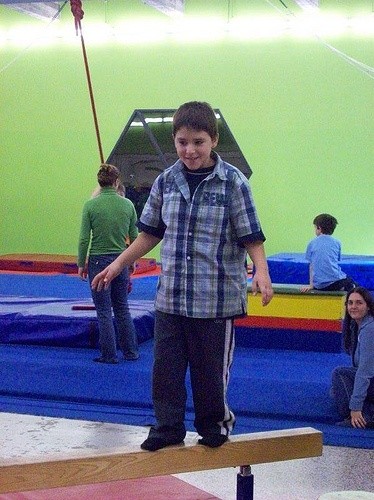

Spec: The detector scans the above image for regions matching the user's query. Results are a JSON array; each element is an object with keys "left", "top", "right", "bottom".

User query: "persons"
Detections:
[
  {"left": 91, "top": 180, "right": 134, "bottom": 294},
  {"left": 75, "top": 163, "right": 140, "bottom": 364},
  {"left": 299, "top": 213, "right": 363, "bottom": 294},
  {"left": 90, "top": 102, "right": 274, "bottom": 453},
  {"left": 331, "top": 285, "right": 374, "bottom": 430}
]
